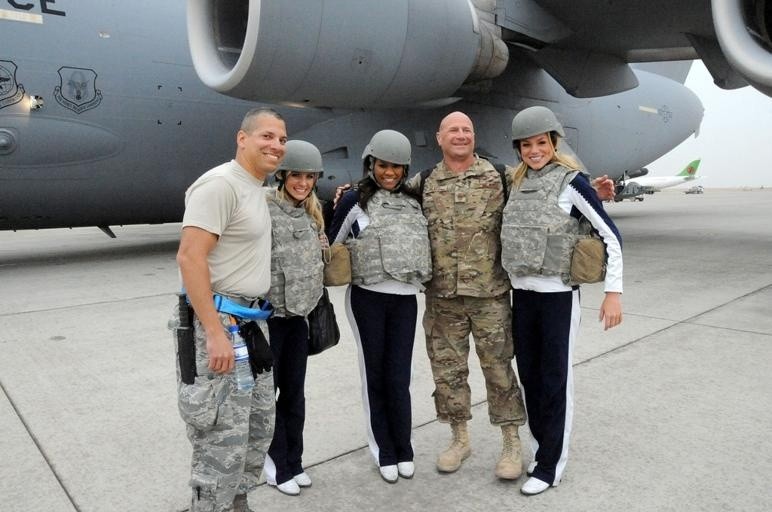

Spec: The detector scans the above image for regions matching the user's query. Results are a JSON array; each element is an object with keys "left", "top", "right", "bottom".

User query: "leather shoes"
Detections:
[
  {"left": 519, "top": 475, "right": 559, "bottom": 493},
  {"left": 381, "top": 465, "right": 399, "bottom": 483},
  {"left": 526, "top": 460, "right": 539, "bottom": 475},
  {"left": 294, "top": 471, "right": 312, "bottom": 487},
  {"left": 267, "top": 476, "right": 299, "bottom": 496},
  {"left": 398, "top": 461, "right": 415, "bottom": 480}
]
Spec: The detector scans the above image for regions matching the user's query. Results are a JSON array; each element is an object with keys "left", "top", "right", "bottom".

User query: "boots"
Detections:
[
  {"left": 437, "top": 421, "right": 472, "bottom": 473},
  {"left": 496, "top": 424, "right": 524, "bottom": 479}
]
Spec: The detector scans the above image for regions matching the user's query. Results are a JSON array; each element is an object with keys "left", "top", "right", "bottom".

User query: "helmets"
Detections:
[
  {"left": 270, "top": 141, "right": 324, "bottom": 179},
  {"left": 361, "top": 130, "right": 412, "bottom": 165},
  {"left": 510, "top": 106, "right": 566, "bottom": 141}
]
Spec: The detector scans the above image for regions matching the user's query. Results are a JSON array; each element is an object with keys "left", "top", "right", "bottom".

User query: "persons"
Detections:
[
  {"left": 327, "top": 129, "right": 432, "bottom": 484},
  {"left": 500, "top": 106, "right": 623, "bottom": 496},
  {"left": 262, "top": 139, "right": 340, "bottom": 496},
  {"left": 334, "top": 111, "right": 617, "bottom": 480},
  {"left": 168, "top": 108, "right": 325, "bottom": 512}
]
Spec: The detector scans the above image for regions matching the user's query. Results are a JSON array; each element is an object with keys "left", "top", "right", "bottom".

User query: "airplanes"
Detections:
[
  {"left": 613, "top": 159, "right": 702, "bottom": 202},
  {"left": 0, "top": 1, "right": 770, "bottom": 239}
]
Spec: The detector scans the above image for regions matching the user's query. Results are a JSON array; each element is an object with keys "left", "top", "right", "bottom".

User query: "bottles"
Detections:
[{"left": 229, "top": 325, "right": 255, "bottom": 393}]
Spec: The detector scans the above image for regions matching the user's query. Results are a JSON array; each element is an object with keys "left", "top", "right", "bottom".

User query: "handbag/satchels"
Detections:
[
  {"left": 241, "top": 320, "right": 275, "bottom": 375},
  {"left": 311, "top": 287, "right": 339, "bottom": 355}
]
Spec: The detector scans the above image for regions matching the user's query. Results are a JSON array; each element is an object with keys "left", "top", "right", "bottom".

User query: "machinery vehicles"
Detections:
[{"left": 685, "top": 185, "right": 704, "bottom": 194}]
